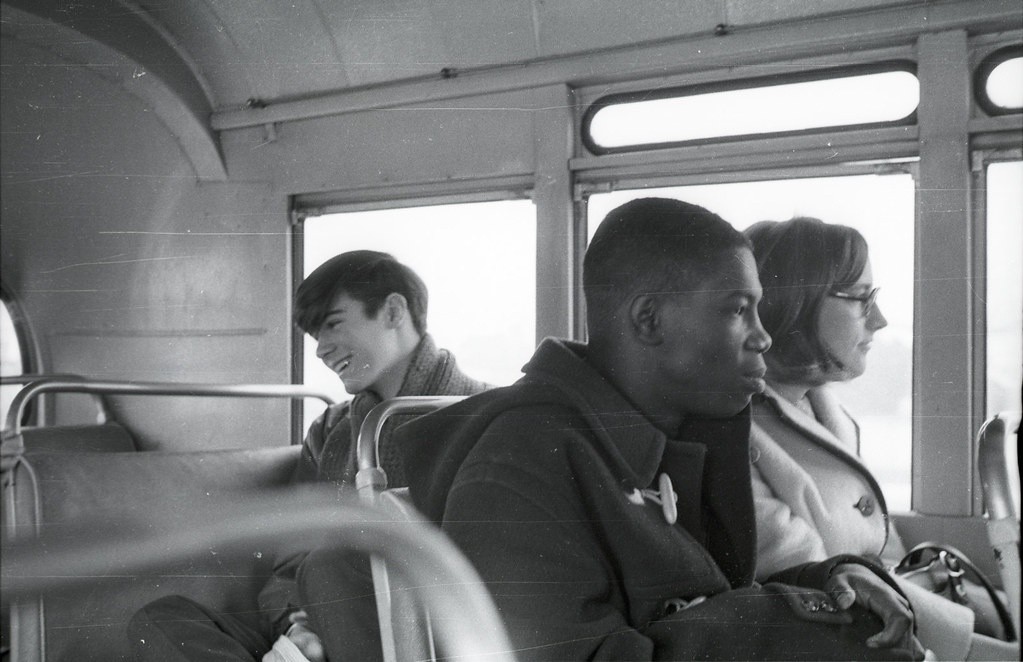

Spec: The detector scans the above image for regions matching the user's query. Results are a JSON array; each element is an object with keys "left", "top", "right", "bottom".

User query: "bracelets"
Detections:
[{"left": 285, "top": 623, "right": 298, "bottom": 636}]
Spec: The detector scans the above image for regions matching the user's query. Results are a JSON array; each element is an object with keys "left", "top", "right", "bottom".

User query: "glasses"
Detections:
[{"left": 828, "top": 288, "right": 880, "bottom": 316}]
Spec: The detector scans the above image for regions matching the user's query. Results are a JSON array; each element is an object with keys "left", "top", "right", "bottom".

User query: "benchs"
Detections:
[{"left": 1, "top": 378, "right": 1021, "bottom": 660}]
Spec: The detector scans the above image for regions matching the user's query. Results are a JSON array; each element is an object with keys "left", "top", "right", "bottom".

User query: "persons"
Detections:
[
  {"left": 741, "top": 217, "right": 1020, "bottom": 662},
  {"left": 395, "top": 198, "right": 925, "bottom": 661},
  {"left": 127, "top": 250, "right": 493, "bottom": 662}
]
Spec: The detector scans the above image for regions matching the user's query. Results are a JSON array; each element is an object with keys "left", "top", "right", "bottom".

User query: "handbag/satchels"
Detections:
[{"left": 888, "top": 540, "right": 1017, "bottom": 643}]
